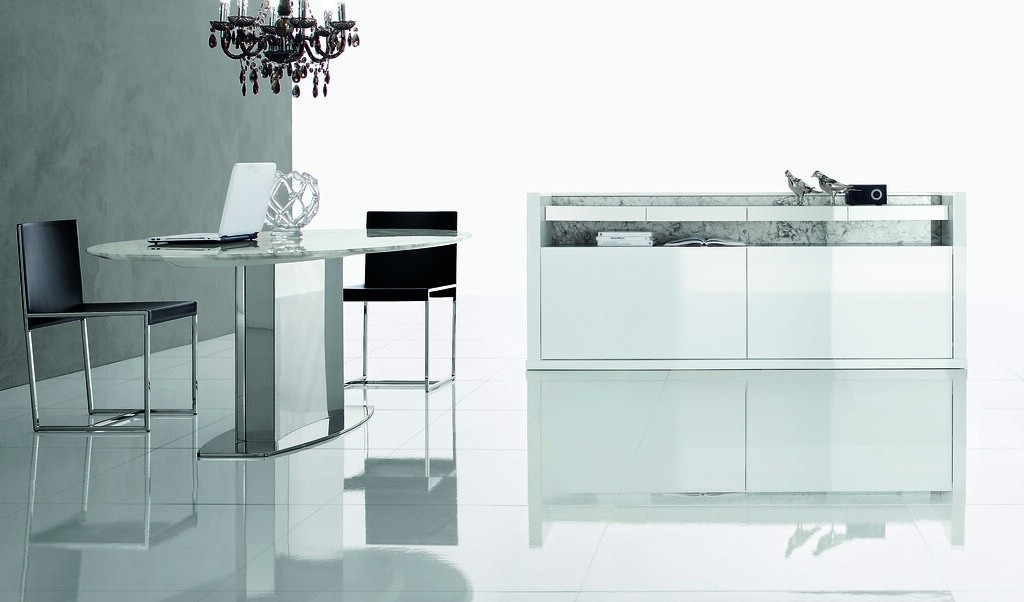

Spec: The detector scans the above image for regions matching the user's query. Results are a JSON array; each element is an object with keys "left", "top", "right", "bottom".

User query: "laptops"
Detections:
[{"left": 146, "top": 162, "right": 277, "bottom": 244}]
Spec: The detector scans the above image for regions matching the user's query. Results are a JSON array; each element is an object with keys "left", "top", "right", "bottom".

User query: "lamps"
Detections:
[{"left": 209, "top": 0, "right": 360, "bottom": 98}]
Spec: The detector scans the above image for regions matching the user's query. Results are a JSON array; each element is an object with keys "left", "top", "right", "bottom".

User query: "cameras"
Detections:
[{"left": 845, "top": 185, "right": 886, "bottom": 206}]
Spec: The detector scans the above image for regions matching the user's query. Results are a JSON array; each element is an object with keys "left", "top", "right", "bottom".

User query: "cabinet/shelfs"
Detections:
[{"left": 526, "top": 193, "right": 967, "bottom": 368}]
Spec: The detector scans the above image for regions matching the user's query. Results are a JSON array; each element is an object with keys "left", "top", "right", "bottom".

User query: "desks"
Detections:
[{"left": 85, "top": 229, "right": 469, "bottom": 459}]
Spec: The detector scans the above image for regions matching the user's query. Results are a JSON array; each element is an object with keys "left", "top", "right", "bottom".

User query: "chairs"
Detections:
[
  {"left": 343, "top": 211, "right": 458, "bottom": 393},
  {"left": 17, "top": 219, "right": 198, "bottom": 431}
]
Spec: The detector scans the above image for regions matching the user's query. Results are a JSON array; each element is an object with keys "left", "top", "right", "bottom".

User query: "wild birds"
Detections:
[
  {"left": 811, "top": 171, "right": 862, "bottom": 205},
  {"left": 785, "top": 170, "right": 823, "bottom": 206}
]
